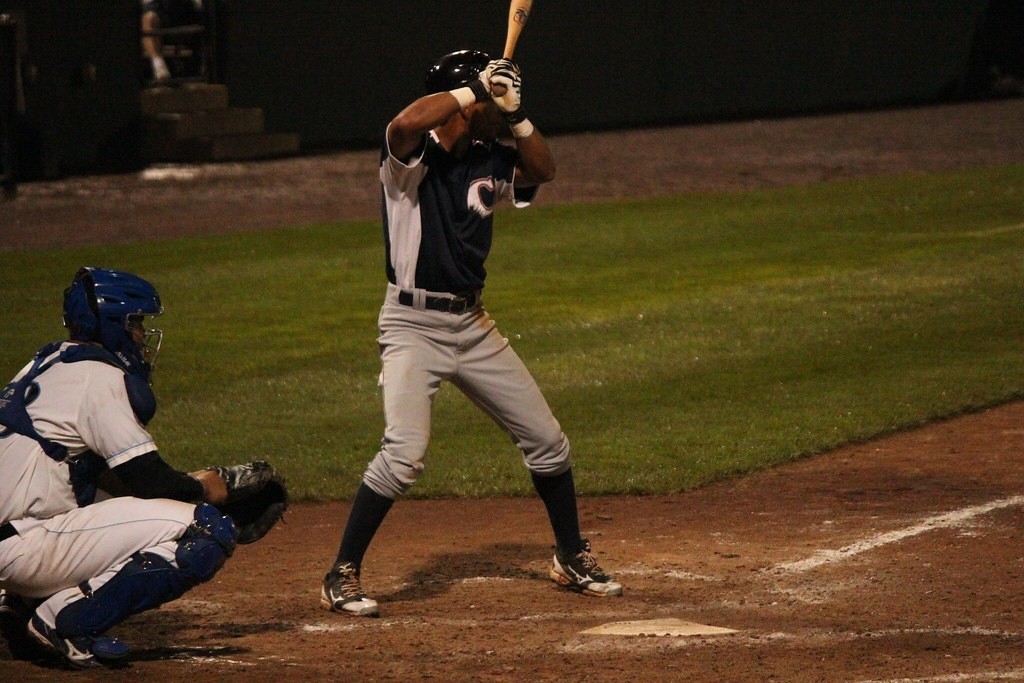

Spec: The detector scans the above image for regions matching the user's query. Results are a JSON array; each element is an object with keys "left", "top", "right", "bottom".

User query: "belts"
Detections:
[
  {"left": 399, "top": 292, "right": 480, "bottom": 313},
  {"left": 0, "top": 520, "right": 19, "bottom": 544}
]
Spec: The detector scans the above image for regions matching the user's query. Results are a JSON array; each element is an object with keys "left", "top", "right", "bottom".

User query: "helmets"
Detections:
[
  {"left": 61, "top": 266, "right": 164, "bottom": 384},
  {"left": 424, "top": 49, "right": 492, "bottom": 91}
]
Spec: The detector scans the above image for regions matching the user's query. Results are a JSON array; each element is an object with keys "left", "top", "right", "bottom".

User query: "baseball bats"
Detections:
[{"left": 491, "top": 1, "right": 533, "bottom": 102}]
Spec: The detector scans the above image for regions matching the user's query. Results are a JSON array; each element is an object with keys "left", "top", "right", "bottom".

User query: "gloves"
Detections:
[{"left": 451, "top": 59, "right": 534, "bottom": 137}]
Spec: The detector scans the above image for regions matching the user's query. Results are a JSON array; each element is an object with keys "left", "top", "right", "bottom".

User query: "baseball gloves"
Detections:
[{"left": 207, "top": 457, "right": 291, "bottom": 550}]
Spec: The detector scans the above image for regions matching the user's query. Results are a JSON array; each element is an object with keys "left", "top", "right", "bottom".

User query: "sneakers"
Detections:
[
  {"left": 549, "top": 537, "right": 621, "bottom": 596},
  {"left": 28, "top": 615, "right": 130, "bottom": 669},
  {"left": 0, "top": 590, "right": 36, "bottom": 618},
  {"left": 320, "top": 559, "right": 379, "bottom": 615}
]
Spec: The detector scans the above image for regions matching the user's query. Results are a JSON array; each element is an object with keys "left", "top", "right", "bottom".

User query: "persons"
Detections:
[
  {"left": 320, "top": 50, "right": 623, "bottom": 618},
  {"left": 0, "top": 267, "right": 240, "bottom": 669},
  {"left": 136, "top": 1, "right": 208, "bottom": 84}
]
